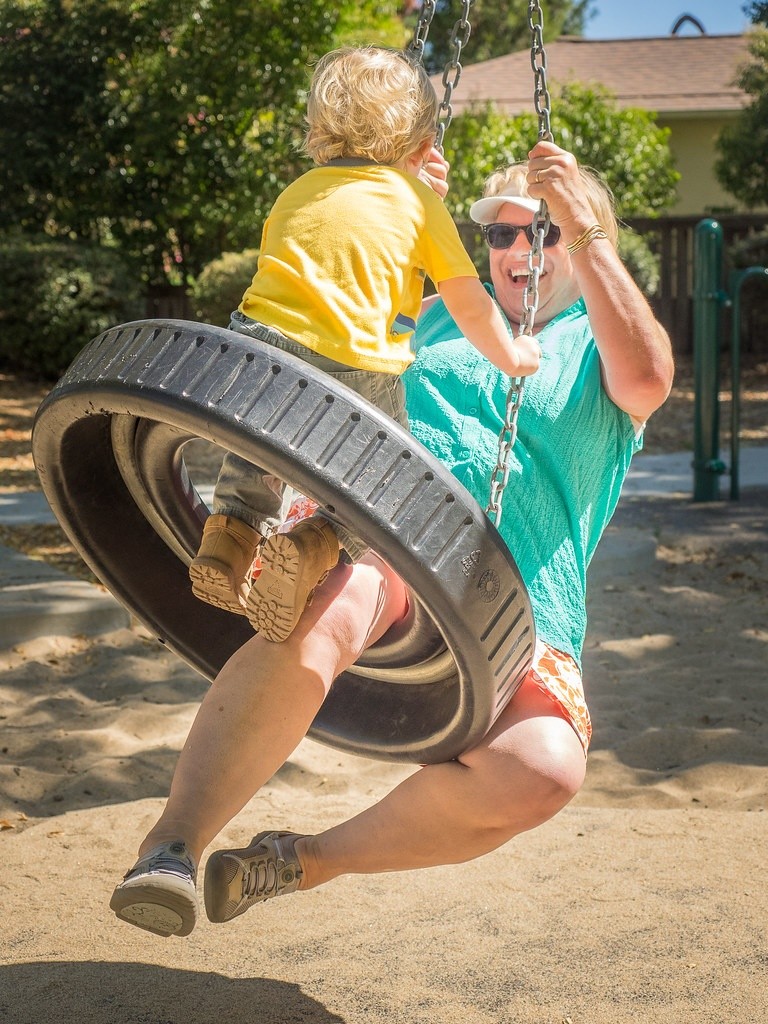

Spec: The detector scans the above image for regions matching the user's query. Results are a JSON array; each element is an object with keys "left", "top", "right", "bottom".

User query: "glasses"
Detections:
[{"left": 478, "top": 221, "right": 560, "bottom": 249}]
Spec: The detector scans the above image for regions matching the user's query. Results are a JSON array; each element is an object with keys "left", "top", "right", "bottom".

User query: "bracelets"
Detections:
[{"left": 565, "top": 222, "right": 609, "bottom": 257}]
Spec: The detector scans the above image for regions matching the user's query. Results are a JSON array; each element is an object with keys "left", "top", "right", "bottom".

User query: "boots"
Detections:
[
  {"left": 242, "top": 518, "right": 340, "bottom": 642},
  {"left": 189, "top": 513, "right": 262, "bottom": 617}
]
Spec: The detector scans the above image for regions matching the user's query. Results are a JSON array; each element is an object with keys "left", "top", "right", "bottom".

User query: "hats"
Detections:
[{"left": 468, "top": 193, "right": 554, "bottom": 225}]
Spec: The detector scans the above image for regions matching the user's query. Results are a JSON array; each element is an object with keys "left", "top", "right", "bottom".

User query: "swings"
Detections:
[{"left": 31, "top": 0, "right": 558, "bottom": 765}]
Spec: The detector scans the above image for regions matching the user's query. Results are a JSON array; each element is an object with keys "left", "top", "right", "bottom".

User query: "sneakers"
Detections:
[
  {"left": 112, "top": 843, "right": 201, "bottom": 940},
  {"left": 203, "top": 830, "right": 306, "bottom": 927}
]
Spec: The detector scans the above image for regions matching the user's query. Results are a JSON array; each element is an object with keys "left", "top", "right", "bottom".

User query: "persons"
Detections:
[
  {"left": 107, "top": 140, "right": 674, "bottom": 937},
  {"left": 188, "top": 41, "right": 540, "bottom": 649}
]
